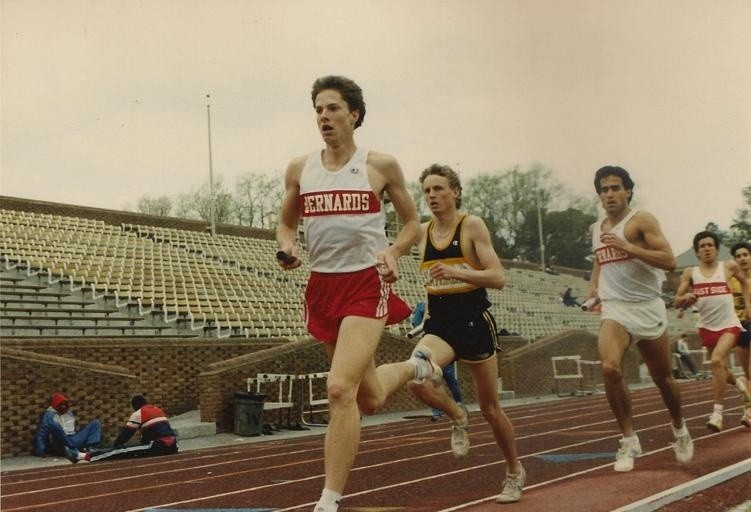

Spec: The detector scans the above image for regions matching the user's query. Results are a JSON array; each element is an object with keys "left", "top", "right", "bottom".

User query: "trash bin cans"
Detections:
[{"left": 232, "top": 391, "right": 268, "bottom": 438}]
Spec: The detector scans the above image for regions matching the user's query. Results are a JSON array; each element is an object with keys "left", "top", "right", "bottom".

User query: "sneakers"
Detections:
[
  {"left": 62, "top": 446, "right": 82, "bottom": 464},
  {"left": 409, "top": 343, "right": 442, "bottom": 389},
  {"left": 613, "top": 435, "right": 643, "bottom": 473},
  {"left": 738, "top": 374, "right": 751, "bottom": 401},
  {"left": 706, "top": 415, "right": 723, "bottom": 434},
  {"left": 741, "top": 408, "right": 751, "bottom": 429},
  {"left": 449, "top": 411, "right": 471, "bottom": 460},
  {"left": 667, "top": 424, "right": 695, "bottom": 464},
  {"left": 495, "top": 460, "right": 528, "bottom": 502}
]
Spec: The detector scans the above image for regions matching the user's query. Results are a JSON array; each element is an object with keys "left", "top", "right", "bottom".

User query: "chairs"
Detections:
[
  {"left": 0, "top": 207, "right": 119, "bottom": 306},
  {"left": 120, "top": 222, "right": 696, "bottom": 337}
]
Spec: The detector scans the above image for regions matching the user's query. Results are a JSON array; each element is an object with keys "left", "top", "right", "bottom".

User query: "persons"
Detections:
[
  {"left": 113, "top": 396, "right": 178, "bottom": 457},
  {"left": 34, "top": 393, "right": 102, "bottom": 463},
  {"left": 276, "top": 76, "right": 442, "bottom": 512},
  {"left": 407, "top": 163, "right": 525, "bottom": 504},
  {"left": 729, "top": 243, "right": 751, "bottom": 427},
  {"left": 672, "top": 232, "right": 750, "bottom": 432},
  {"left": 562, "top": 287, "right": 582, "bottom": 308},
  {"left": 433, "top": 360, "right": 462, "bottom": 420},
  {"left": 585, "top": 166, "right": 693, "bottom": 472}
]
